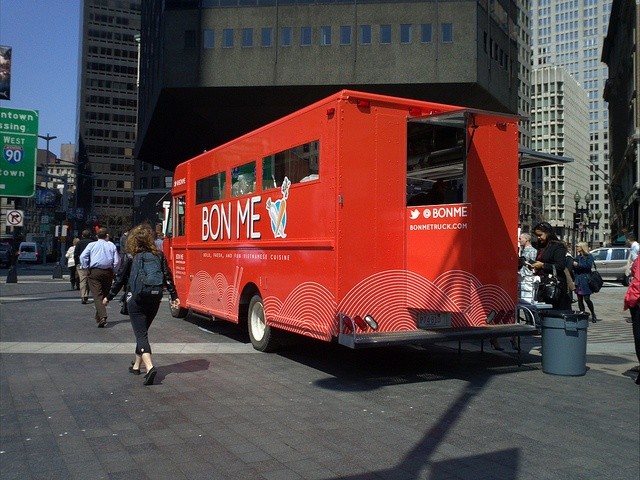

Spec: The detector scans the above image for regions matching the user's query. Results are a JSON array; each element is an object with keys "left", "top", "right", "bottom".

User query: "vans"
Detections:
[{"left": 17, "top": 242, "right": 38, "bottom": 263}]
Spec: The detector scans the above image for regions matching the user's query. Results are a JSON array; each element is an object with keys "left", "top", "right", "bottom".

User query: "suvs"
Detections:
[{"left": 588, "top": 248, "right": 632, "bottom": 286}]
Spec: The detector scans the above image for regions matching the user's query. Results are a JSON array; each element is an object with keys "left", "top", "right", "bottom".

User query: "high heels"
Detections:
[
  {"left": 489, "top": 338, "right": 504, "bottom": 350},
  {"left": 510, "top": 339, "right": 522, "bottom": 351}
]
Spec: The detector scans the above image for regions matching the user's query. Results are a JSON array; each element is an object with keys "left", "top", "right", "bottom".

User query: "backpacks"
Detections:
[{"left": 127, "top": 251, "right": 164, "bottom": 302}]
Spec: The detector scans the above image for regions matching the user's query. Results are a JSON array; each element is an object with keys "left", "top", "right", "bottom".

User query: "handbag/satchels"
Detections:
[
  {"left": 542, "top": 264, "right": 562, "bottom": 301},
  {"left": 118, "top": 286, "right": 129, "bottom": 315},
  {"left": 586, "top": 261, "right": 603, "bottom": 292}
]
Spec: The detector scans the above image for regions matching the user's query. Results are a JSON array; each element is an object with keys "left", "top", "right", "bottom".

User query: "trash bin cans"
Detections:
[{"left": 538, "top": 309, "right": 590, "bottom": 376}]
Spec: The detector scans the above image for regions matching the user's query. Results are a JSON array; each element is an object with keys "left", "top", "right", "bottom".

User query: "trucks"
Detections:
[{"left": 162, "top": 87, "right": 537, "bottom": 352}]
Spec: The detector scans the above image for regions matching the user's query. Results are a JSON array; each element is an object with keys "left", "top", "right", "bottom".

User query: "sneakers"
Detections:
[
  {"left": 129, "top": 361, "right": 140, "bottom": 375},
  {"left": 144, "top": 366, "right": 157, "bottom": 385}
]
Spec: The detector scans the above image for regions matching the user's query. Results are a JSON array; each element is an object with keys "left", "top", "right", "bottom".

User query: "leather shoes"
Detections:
[
  {"left": 592, "top": 313, "right": 596, "bottom": 322},
  {"left": 81, "top": 298, "right": 85, "bottom": 304},
  {"left": 97, "top": 318, "right": 106, "bottom": 327}
]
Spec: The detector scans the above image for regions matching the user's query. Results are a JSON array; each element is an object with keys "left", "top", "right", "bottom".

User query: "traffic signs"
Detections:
[{"left": 1, "top": 108, "right": 38, "bottom": 198}]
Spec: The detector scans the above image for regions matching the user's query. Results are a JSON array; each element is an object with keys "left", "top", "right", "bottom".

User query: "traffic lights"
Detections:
[{"left": 0, "top": 45, "right": 12, "bottom": 98}]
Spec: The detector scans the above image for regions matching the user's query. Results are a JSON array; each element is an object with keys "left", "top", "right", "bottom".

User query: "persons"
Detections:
[
  {"left": 531, "top": 222, "right": 567, "bottom": 312},
  {"left": 570, "top": 241, "right": 598, "bottom": 323},
  {"left": 119, "top": 226, "right": 131, "bottom": 265},
  {"left": 623, "top": 254, "right": 639, "bottom": 385},
  {"left": 80, "top": 227, "right": 121, "bottom": 327},
  {"left": 74, "top": 229, "right": 92, "bottom": 306},
  {"left": 66, "top": 237, "right": 80, "bottom": 292},
  {"left": 623, "top": 233, "right": 640, "bottom": 284},
  {"left": 519, "top": 233, "right": 538, "bottom": 276},
  {"left": 101, "top": 225, "right": 180, "bottom": 385}
]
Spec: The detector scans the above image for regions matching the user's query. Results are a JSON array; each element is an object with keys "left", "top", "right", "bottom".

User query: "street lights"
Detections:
[{"left": 572, "top": 191, "right": 602, "bottom": 258}]
